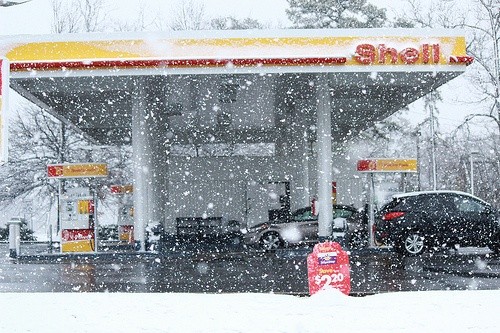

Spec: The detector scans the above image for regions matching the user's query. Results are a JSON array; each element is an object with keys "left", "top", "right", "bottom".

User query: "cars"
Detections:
[
  {"left": 242, "top": 205, "right": 369, "bottom": 253},
  {"left": 374, "top": 190, "right": 500, "bottom": 256}
]
